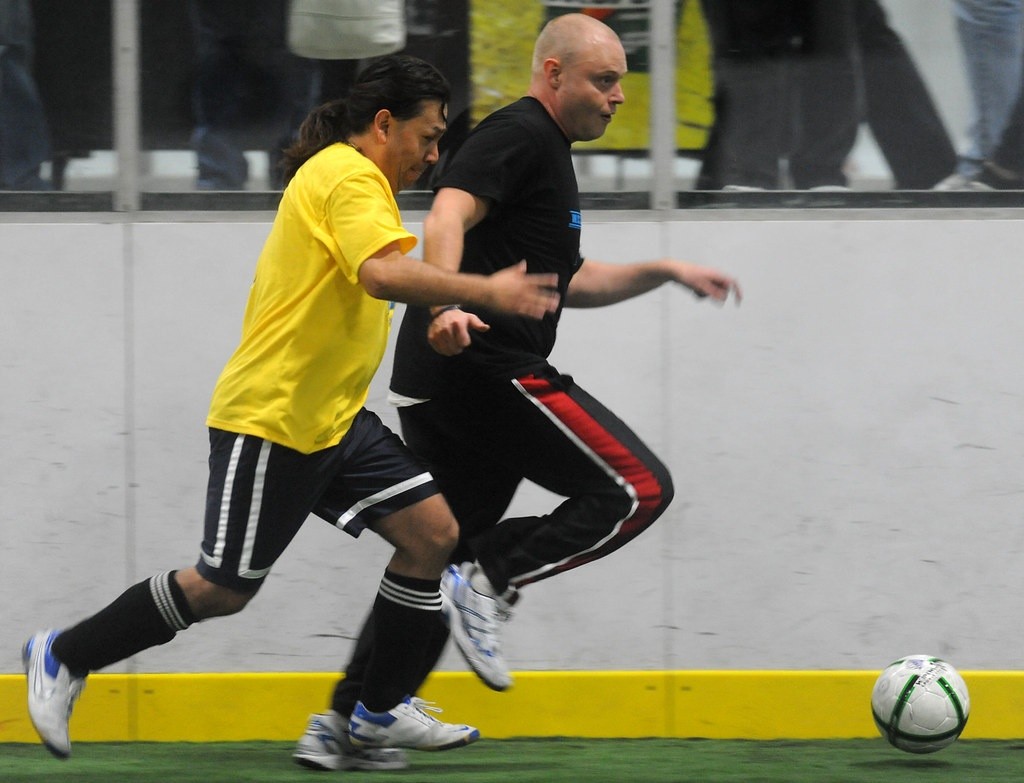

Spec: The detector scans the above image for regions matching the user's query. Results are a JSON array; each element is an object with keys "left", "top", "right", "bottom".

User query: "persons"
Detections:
[
  {"left": 22, "top": 49, "right": 481, "bottom": 754},
  {"left": 290, "top": 11, "right": 743, "bottom": 774},
  {"left": 174, "top": 0, "right": 1024, "bottom": 192}
]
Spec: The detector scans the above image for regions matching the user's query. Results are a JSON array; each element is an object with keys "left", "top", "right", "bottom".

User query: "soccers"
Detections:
[{"left": 869, "top": 656, "right": 970, "bottom": 752}]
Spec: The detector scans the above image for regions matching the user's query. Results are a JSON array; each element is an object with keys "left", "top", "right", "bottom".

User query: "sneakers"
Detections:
[
  {"left": 442, "top": 561, "right": 512, "bottom": 692},
  {"left": 20, "top": 629, "right": 90, "bottom": 756},
  {"left": 347, "top": 697, "right": 481, "bottom": 753},
  {"left": 291, "top": 711, "right": 407, "bottom": 773}
]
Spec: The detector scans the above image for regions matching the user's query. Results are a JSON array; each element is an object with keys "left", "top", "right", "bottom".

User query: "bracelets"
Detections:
[{"left": 431, "top": 305, "right": 459, "bottom": 319}]
